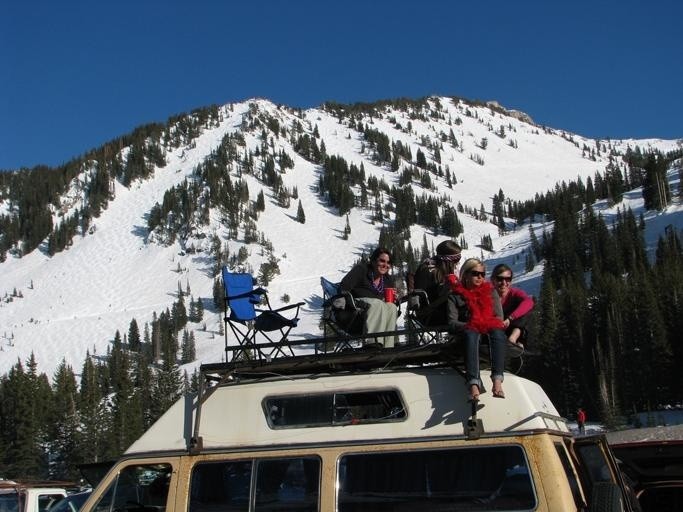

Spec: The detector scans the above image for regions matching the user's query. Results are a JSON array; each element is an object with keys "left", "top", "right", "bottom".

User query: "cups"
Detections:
[
  {"left": 448, "top": 274, "right": 457, "bottom": 289},
  {"left": 384, "top": 288, "right": 396, "bottom": 302}
]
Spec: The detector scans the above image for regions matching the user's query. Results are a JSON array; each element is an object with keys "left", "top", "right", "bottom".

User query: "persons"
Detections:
[
  {"left": 447, "top": 258, "right": 507, "bottom": 401},
  {"left": 332, "top": 247, "right": 404, "bottom": 355},
  {"left": 577, "top": 408, "right": 586, "bottom": 436},
  {"left": 491, "top": 265, "right": 535, "bottom": 358},
  {"left": 413, "top": 241, "right": 481, "bottom": 362}
]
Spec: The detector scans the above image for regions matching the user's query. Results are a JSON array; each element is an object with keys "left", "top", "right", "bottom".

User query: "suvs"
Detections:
[{"left": 1, "top": 481, "right": 75, "bottom": 512}]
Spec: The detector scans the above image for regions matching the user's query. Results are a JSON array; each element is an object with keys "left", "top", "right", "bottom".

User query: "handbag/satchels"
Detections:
[{"left": 458, "top": 304, "right": 471, "bottom": 322}]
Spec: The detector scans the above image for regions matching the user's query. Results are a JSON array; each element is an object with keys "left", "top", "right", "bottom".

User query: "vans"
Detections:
[{"left": 41, "top": 337, "right": 641, "bottom": 512}]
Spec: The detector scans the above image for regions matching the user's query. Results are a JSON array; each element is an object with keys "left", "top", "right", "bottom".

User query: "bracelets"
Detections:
[{"left": 507, "top": 316, "right": 513, "bottom": 325}]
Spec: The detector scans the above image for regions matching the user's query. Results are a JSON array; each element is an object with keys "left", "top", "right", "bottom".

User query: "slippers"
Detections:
[
  {"left": 492, "top": 389, "right": 505, "bottom": 398},
  {"left": 466, "top": 393, "right": 480, "bottom": 403}
]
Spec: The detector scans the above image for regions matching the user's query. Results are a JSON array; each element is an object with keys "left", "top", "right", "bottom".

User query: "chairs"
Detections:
[
  {"left": 320, "top": 276, "right": 443, "bottom": 353},
  {"left": 219, "top": 265, "right": 305, "bottom": 366}
]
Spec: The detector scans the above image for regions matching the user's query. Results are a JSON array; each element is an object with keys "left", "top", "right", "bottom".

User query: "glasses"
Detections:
[
  {"left": 495, "top": 276, "right": 512, "bottom": 282},
  {"left": 471, "top": 271, "right": 485, "bottom": 278}
]
